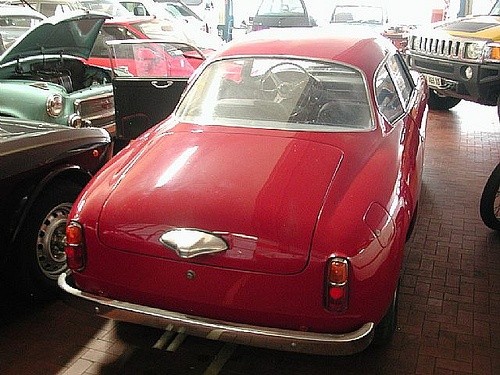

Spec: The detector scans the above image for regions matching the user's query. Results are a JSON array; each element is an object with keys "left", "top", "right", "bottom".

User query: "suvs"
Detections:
[{"left": 407, "top": 0, "right": 500, "bottom": 126}]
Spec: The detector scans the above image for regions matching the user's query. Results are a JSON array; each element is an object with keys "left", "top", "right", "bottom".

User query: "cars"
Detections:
[
  {"left": 244, "top": 1, "right": 316, "bottom": 31},
  {"left": 2, "top": 2, "right": 388, "bottom": 39},
  {"left": 1, "top": 13, "right": 217, "bottom": 76},
  {"left": 0, "top": 73, "right": 73, "bottom": 126},
  {"left": 59, "top": 24, "right": 429, "bottom": 356},
  {"left": 0, "top": 116, "right": 112, "bottom": 294}
]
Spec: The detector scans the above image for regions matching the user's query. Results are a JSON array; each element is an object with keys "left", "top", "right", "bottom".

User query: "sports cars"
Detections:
[{"left": 329, "top": 1, "right": 388, "bottom": 24}]
